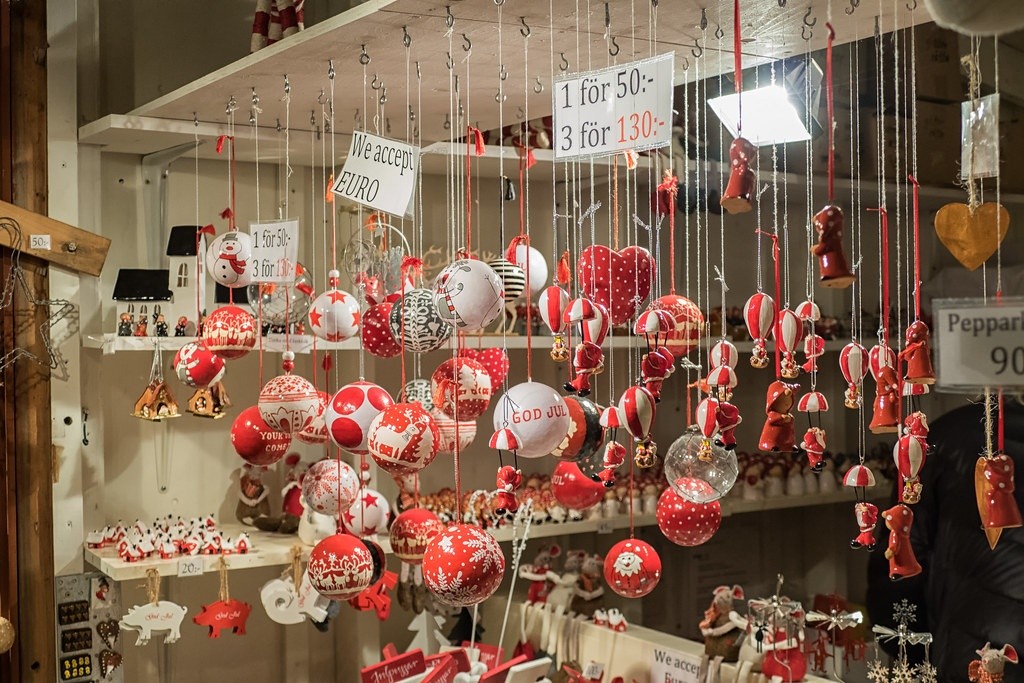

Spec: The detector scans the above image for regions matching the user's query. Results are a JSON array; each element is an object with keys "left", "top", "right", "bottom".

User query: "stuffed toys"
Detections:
[
  {"left": 237, "top": 454, "right": 316, "bottom": 535},
  {"left": 517, "top": 543, "right": 809, "bottom": 683}
]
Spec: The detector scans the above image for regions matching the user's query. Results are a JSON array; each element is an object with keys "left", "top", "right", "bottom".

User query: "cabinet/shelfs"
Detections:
[{"left": 77, "top": 112, "right": 1024, "bottom": 584}]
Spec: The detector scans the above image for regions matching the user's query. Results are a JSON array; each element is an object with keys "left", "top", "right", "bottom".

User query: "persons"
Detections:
[{"left": 865, "top": 392, "right": 1024, "bottom": 683}]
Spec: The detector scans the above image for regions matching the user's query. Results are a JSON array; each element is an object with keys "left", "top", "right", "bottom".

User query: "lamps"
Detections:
[{"left": 705, "top": 58, "right": 825, "bottom": 150}]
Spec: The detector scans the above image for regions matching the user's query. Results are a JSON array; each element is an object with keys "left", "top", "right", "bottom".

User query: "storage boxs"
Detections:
[{"left": 700, "top": 25, "right": 1024, "bottom": 192}]
